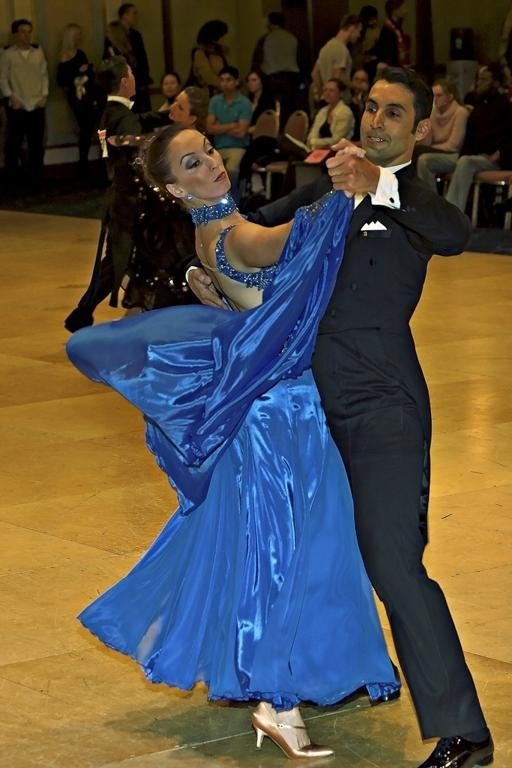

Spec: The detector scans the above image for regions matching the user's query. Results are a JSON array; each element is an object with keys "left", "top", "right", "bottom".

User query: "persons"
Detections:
[
  {"left": 0, "top": 19, "right": 49, "bottom": 207},
  {"left": 64, "top": 55, "right": 175, "bottom": 332},
  {"left": 243, "top": 63, "right": 495, "bottom": 767},
  {"left": 106, "top": 87, "right": 212, "bottom": 312},
  {"left": 65, "top": 124, "right": 402, "bottom": 760}
]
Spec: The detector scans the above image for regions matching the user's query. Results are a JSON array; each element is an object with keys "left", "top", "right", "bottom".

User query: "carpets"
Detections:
[{"left": 0, "top": 183, "right": 511, "bottom": 258}]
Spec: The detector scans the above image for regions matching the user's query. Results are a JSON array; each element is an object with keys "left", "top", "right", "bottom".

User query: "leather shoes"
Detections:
[
  {"left": 65, "top": 309, "right": 94, "bottom": 332},
  {"left": 418, "top": 728, "right": 495, "bottom": 767}
]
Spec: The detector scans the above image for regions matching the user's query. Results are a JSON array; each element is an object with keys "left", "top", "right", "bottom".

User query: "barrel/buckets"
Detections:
[{"left": 450, "top": 26, "right": 473, "bottom": 59}]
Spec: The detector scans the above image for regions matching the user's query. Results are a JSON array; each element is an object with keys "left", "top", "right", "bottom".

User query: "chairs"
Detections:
[
  {"left": 471, "top": 170, "right": 510, "bottom": 229},
  {"left": 226, "top": 94, "right": 310, "bottom": 196}
]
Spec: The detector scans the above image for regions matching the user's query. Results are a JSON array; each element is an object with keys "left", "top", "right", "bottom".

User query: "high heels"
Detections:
[{"left": 251, "top": 702, "right": 335, "bottom": 760}]
